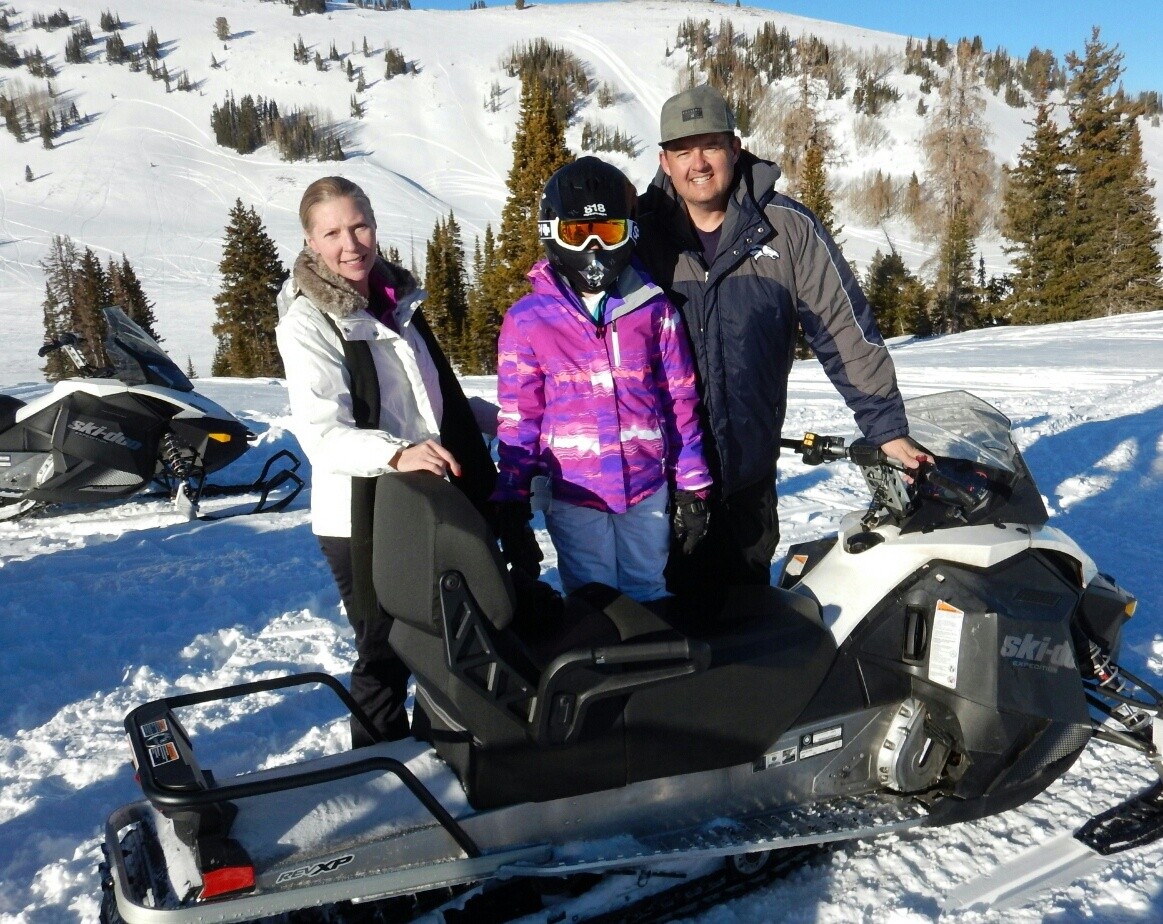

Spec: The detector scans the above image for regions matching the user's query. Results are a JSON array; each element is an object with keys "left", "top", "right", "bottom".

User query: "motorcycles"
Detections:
[
  {"left": 97, "top": 390, "right": 1162, "bottom": 922},
  {"left": 0, "top": 306, "right": 306, "bottom": 526}
]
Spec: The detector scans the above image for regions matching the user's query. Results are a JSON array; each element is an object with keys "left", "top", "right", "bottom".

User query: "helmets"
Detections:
[{"left": 537, "top": 156, "right": 639, "bottom": 292}]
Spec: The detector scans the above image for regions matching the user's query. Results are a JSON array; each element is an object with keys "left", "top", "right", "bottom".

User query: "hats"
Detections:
[{"left": 658, "top": 85, "right": 736, "bottom": 146}]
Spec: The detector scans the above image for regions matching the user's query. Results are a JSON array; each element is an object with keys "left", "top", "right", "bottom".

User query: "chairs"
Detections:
[{"left": 363, "top": 465, "right": 840, "bottom": 799}]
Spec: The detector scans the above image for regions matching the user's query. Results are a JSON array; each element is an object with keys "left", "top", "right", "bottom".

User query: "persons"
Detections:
[
  {"left": 497, "top": 155, "right": 717, "bottom": 601},
  {"left": 632, "top": 84, "right": 939, "bottom": 585},
  {"left": 273, "top": 174, "right": 500, "bottom": 752}
]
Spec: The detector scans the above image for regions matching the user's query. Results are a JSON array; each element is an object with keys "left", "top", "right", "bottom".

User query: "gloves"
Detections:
[
  {"left": 494, "top": 501, "right": 543, "bottom": 579},
  {"left": 673, "top": 495, "right": 712, "bottom": 554}
]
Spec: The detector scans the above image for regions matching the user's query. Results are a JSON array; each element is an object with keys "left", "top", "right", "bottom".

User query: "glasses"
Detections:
[{"left": 536, "top": 216, "right": 640, "bottom": 250}]
